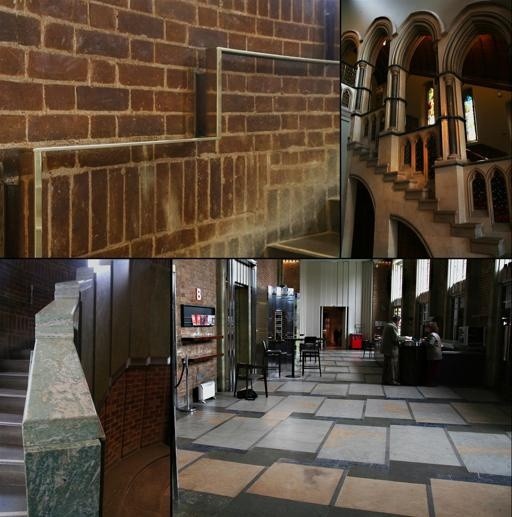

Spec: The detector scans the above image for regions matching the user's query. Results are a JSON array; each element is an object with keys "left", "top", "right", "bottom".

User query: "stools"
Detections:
[{"left": 302, "top": 350, "right": 322, "bottom": 377}]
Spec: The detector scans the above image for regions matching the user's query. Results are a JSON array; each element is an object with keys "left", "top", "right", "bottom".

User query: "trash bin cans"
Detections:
[{"left": 351, "top": 333, "right": 362, "bottom": 349}]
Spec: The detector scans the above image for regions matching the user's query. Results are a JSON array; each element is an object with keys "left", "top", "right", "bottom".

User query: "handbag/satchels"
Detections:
[{"left": 237, "top": 389, "right": 257, "bottom": 400}]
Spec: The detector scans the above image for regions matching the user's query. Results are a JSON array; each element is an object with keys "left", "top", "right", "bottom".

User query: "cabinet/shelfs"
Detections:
[{"left": 181, "top": 335, "right": 226, "bottom": 362}]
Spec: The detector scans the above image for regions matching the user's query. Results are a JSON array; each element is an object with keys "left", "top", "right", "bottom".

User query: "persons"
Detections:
[
  {"left": 380, "top": 315, "right": 400, "bottom": 386},
  {"left": 425, "top": 321, "right": 442, "bottom": 387}
]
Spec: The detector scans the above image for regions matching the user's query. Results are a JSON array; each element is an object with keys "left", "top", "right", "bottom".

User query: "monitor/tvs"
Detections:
[{"left": 180, "top": 304, "right": 215, "bottom": 328}]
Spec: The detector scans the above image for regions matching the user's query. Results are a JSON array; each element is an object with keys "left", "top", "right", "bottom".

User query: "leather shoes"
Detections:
[{"left": 382, "top": 379, "right": 400, "bottom": 385}]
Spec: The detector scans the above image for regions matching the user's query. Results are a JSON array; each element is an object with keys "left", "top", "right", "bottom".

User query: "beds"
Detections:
[{"left": 263, "top": 350, "right": 282, "bottom": 378}]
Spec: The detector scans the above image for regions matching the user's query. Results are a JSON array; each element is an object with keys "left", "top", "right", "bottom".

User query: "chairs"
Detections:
[
  {"left": 234, "top": 361, "right": 268, "bottom": 398},
  {"left": 263, "top": 338, "right": 296, "bottom": 377},
  {"left": 300, "top": 337, "right": 317, "bottom": 362},
  {"left": 363, "top": 341, "right": 376, "bottom": 358}
]
